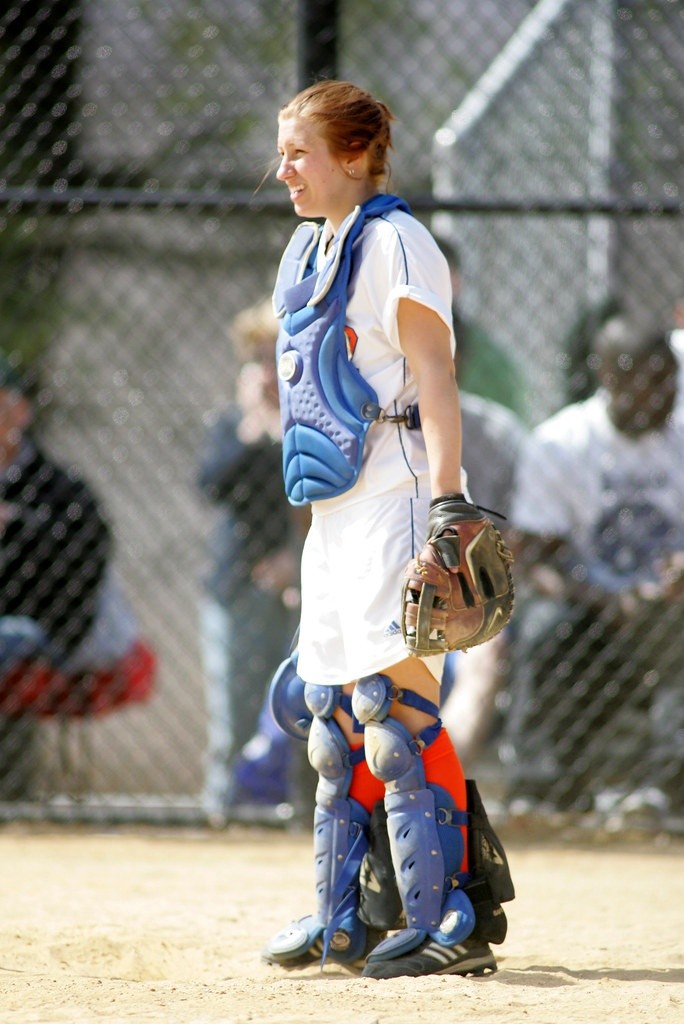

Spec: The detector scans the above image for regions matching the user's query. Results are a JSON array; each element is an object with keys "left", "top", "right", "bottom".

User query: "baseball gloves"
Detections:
[{"left": 401, "top": 495, "right": 520, "bottom": 657}]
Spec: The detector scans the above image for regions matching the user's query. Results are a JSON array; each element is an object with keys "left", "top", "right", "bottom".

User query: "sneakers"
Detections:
[
  {"left": 362, "top": 932, "right": 498, "bottom": 979},
  {"left": 263, "top": 929, "right": 389, "bottom": 973}
]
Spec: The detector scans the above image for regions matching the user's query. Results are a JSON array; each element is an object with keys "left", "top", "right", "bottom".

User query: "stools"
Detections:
[{"left": 0, "top": 640, "right": 155, "bottom": 823}]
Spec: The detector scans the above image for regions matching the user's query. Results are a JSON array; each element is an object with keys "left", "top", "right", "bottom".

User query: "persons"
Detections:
[
  {"left": 261, "top": 79, "right": 513, "bottom": 985},
  {"left": 0, "top": 236, "right": 684, "bottom": 819}
]
